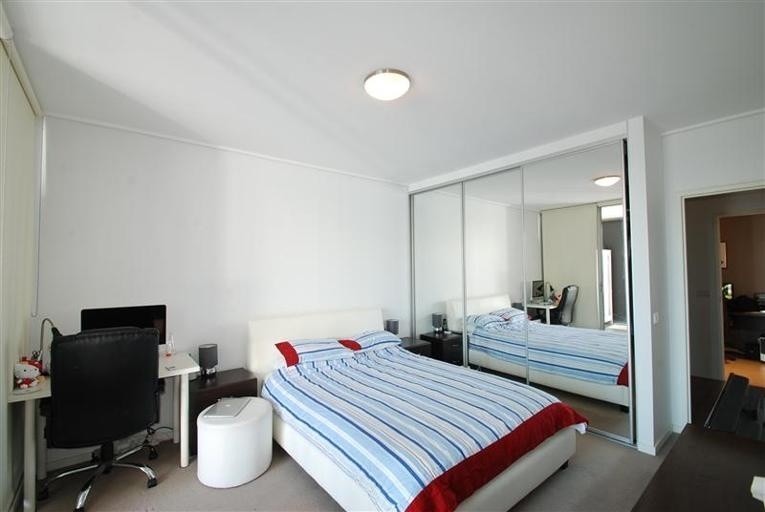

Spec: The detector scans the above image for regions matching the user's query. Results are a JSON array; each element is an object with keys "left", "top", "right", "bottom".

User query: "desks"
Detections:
[
  {"left": 522, "top": 302, "right": 558, "bottom": 324},
  {"left": 8, "top": 352, "right": 200, "bottom": 512},
  {"left": 630, "top": 424, "right": 765, "bottom": 512}
]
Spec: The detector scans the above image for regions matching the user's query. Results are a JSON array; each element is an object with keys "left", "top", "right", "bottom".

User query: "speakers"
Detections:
[
  {"left": 198, "top": 344, "right": 218, "bottom": 369},
  {"left": 431, "top": 312, "right": 444, "bottom": 331},
  {"left": 386, "top": 318, "right": 400, "bottom": 335}
]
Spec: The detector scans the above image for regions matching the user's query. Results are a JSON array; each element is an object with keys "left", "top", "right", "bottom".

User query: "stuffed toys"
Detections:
[{"left": 14, "top": 360, "right": 42, "bottom": 389}]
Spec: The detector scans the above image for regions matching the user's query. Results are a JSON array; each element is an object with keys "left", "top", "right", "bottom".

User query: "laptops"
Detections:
[{"left": 203, "top": 396, "right": 251, "bottom": 418}]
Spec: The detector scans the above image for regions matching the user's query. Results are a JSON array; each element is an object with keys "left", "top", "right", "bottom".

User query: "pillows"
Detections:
[
  {"left": 340, "top": 329, "right": 402, "bottom": 354},
  {"left": 490, "top": 308, "right": 532, "bottom": 324},
  {"left": 467, "top": 314, "right": 506, "bottom": 330},
  {"left": 274, "top": 340, "right": 353, "bottom": 369}
]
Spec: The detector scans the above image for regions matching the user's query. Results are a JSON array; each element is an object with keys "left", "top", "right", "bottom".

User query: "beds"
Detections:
[
  {"left": 446, "top": 295, "right": 629, "bottom": 412},
  {"left": 246, "top": 308, "right": 588, "bottom": 512}
]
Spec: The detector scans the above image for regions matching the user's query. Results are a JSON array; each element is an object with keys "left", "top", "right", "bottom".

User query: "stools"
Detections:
[{"left": 196, "top": 397, "right": 273, "bottom": 489}]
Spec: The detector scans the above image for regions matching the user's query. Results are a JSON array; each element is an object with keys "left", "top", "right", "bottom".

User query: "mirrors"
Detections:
[{"left": 411, "top": 140, "right": 646, "bottom": 444}]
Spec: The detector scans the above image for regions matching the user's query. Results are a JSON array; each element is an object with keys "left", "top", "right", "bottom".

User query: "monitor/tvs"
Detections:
[
  {"left": 80, "top": 304, "right": 167, "bottom": 355},
  {"left": 532, "top": 280, "right": 545, "bottom": 299}
]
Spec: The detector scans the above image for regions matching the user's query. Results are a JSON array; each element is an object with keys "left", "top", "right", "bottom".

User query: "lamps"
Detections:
[
  {"left": 38, "top": 318, "right": 62, "bottom": 375},
  {"left": 364, "top": 68, "right": 410, "bottom": 101},
  {"left": 387, "top": 319, "right": 399, "bottom": 335},
  {"left": 594, "top": 175, "right": 621, "bottom": 187},
  {"left": 199, "top": 344, "right": 218, "bottom": 379},
  {"left": 432, "top": 314, "right": 443, "bottom": 334}
]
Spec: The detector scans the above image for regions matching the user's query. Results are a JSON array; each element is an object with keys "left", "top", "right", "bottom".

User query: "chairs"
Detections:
[
  {"left": 542, "top": 285, "right": 579, "bottom": 326},
  {"left": 39, "top": 327, "right": 165, "bottom": 512}
]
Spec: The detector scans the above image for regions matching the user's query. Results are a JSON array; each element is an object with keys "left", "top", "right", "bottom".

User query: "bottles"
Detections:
[{"left": 164, "top": 332, "right": 176, "bottom": 368}]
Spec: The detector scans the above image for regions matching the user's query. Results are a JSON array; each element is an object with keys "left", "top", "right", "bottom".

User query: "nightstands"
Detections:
[
  {"left": 421, "top": 332, "right": 469, "bottom": 363},
  {"left": 401, "top": 337, "right": 432, "bottom": 358},
  {"left": 180, "top": 368, "right": 258, "bottom": 457}
]
